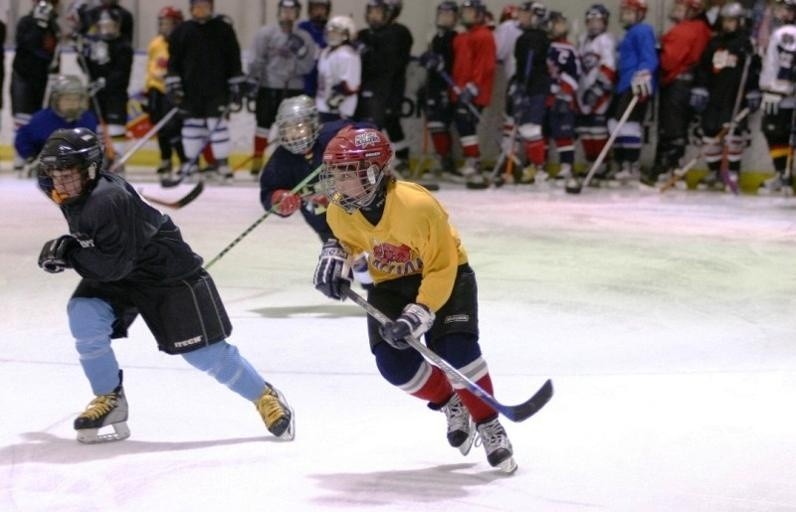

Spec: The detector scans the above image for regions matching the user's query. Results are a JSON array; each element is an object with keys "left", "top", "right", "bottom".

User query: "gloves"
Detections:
[
  {"left": 271, "top": 189, "right": 303, "bottom": 217},
  {"left": 165, "top": 73, "right": 185, "bottom": 106},
  {"left": 745, "top": 89, "right": 781, "bottom": 118},
  {"left": 377, "top": 302, "right": 436, "bottom": 350},
  {"left": 327, "top": 91, "right": 347, "bottom": 109},
  {"left": 310, "top": 238, "right": 356, "bottom": 302},
  {"left": 227, "top": 73, "right": 258, "bottom": 104},
  {"left": 32, "top": 0, "right": 55, "bottom": 30},
  {"left": 457, "top": 89, "right": 474, "bottom": 108},
  {"left": 37, "top": 234, "right": 78, "bottom": 274},
  {"left": 419, "top": 51, "right": 443, "bottom": 76},
  {"left": 688, "top": 85, "right": 708, "bottom": 111},
  {"left": 504, "top": 69, "right": 652, "bottom": 119}
]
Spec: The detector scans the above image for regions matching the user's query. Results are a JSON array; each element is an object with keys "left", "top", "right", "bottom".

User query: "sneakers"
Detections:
[
  {"left": 72, "top": 368, "right": 130, "bottom": 431},
  {"left": 254, "top": 380, "right": 292, "bottom": 437},
  {"left": 423, "top": 158, "right": 689, "bottom": 190}
]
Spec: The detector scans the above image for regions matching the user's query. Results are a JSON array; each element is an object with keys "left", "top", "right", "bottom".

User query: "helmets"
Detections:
[
  {"left": 189, "top": 0, "right": 216, "bottom": 21},
  {"left": 274, "top": 93, "right": 322, "bottom": 157},
  {"left": 320, "top": 124, "right": 399, "bottom": 213},
  {"left": 323, "top": 14, "right": 357, "bottom": 49},
  {"left": 49, "top": 73, "right": 90, "bottom": 123},
  {"left": 36, "top": 125, "right": 104, "bottom": 204},
  {"left": 364, "top": 0, "right": 404, "bottom": 30},
  {"left": 97, "top": 8, "right": 124, "bottom": 37},
  {"left": 275, "top": 0, "right": 303, "bottom": 24},
  {"left": 668, "top": 1, "right": 705, "bottom": 24},
  {"left": 157, "top": 5, "right": 184, "bottom": 37},
  {"left": 305, "top": 0, "right": 333, "bottom": 22},
  {"left": 715, "top": 1, "right": 749, "bottom": 37},
  {"left": 433, "top": 0, "right": 649, "bottom": 41}
]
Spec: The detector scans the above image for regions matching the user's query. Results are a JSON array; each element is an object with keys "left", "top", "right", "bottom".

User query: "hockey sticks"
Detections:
[
  {"left": 137, "top": 180, "right": 205, "bottom": 207},
  {"left": 467, "top": 51, "right": 531, "bottom": 189},
  {"left": 160, "top": 97, "right": 234, "bottom": 186},
  {"left": 440, "top": 71, "right": 537, "bottom": 184},
  {"left": 658, "top": 97, "right": 761, "bottom": 191},
  {"left": 565, "top": 94, "right": 641, "bottom": 194},
  {"left": 720, "top": 55, "right": 752, "bottom": 197},
  {"left": 339, "top": 285, "right": 554, "bottom": 422}
]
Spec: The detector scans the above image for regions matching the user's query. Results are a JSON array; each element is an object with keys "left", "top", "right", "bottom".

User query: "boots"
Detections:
[
  {"left": 724, "top": 170, "right": 739, "bottom": 194},
  {"left": 756, "top": 166, "right": 795, "bottom": 191},
  {"left": 695, "top": 169, "right": 725, "bottom": 190},
  {"left": 155, "top": 158, "right": 236, "bottom": 181},
  {"left": 476, "top": 417, "right": 514, "bottom": 468},
  {"left": 427, "top": 392, "right": 473, "bottom": 448}
]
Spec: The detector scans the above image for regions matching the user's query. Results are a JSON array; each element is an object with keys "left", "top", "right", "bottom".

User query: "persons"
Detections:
[
  {"left": 37, "top": 127, "right": 289, "bottom": 436},
  {"left": 256, "top": 95, "right": 374, "bottom": 245},
  {"left": 314, "top": 130, "right": 514, "bottom": 470}
]
